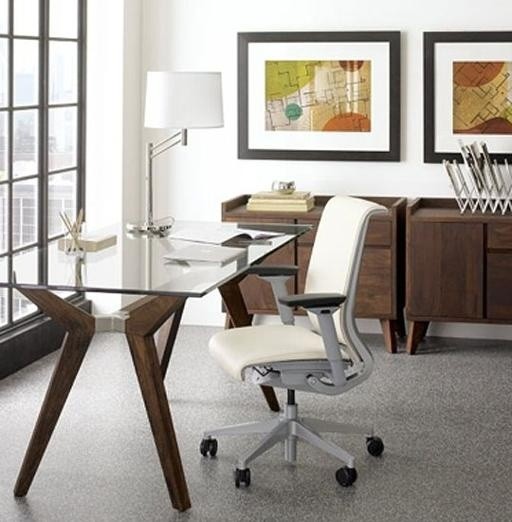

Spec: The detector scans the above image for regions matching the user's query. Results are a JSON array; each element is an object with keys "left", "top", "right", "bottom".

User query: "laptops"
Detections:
[{"left": 164, "top": 245, "right": 249, "bottom": 264}]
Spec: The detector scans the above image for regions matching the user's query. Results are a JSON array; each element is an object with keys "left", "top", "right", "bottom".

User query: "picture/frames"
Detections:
[{"left": 236, "top": 29, "right": 511, "bottom": 166}]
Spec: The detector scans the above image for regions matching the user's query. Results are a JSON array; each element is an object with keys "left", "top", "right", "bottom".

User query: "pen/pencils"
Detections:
[{"left": 59, "top": 206, "right": 85, "bottom": 254}]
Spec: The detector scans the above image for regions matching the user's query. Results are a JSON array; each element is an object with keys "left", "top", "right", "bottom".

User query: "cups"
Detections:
[{"left": 272, "top": 180, "right": 297, "bottom": 194}]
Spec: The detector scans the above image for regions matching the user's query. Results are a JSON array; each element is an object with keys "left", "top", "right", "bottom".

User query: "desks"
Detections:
[{"left": 0, "top": 223, "right": 311, "bottom": 515}]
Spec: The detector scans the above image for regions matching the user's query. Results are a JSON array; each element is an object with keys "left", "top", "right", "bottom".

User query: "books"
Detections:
[
  {"left": 457, "top": 139, "right": 500, "bottom": 196},
  {"left": 246, "top": 192, "right": 316, "bottom": 213},
  {"left": 56, "top": 232, "right": 118, "bottom": 254}
]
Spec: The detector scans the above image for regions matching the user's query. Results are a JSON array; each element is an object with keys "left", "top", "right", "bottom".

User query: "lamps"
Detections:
[{"left": 128, "top": 66, "right": 224, "bottom": 241}]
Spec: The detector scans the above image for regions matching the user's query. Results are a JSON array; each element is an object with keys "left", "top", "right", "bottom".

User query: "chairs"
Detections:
[{"left": 201, "top": 197, "right": 382, "bottom": 487}]
